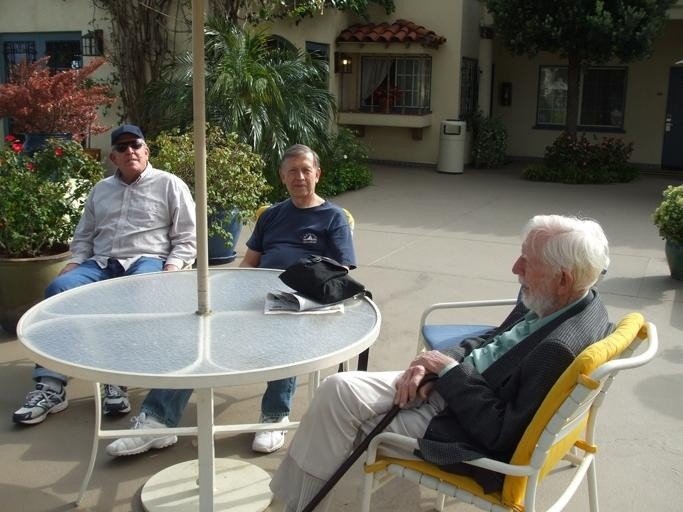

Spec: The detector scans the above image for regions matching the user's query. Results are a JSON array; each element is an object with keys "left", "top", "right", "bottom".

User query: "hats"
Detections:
[{"left": 111, "top": 124, "right": 144, "bottom": 146}]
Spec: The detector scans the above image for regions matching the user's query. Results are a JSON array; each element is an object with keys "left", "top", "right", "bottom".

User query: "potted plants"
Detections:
[
  {"left": 650, "top": 184, "right": 683, "bottom": 280},
  {"left": 0, "top": 55, "right": 120, "bottom": 181},
  {"left": 74, "top": 71, "right": 120, "bottom": 162},
  {"left": 145, "top": 121, "right": 274, "bottom": 261}
]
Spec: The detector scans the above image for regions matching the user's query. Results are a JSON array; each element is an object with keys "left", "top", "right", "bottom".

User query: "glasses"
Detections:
[{"left": 111, "top": 140, "right": 144, "bottom": 153}]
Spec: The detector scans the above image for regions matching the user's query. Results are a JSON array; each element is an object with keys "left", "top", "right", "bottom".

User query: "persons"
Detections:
[
  {"left": 105, "top": 142, "right": 355, "bottom": 458},
  {"left": 270, "top": 214, "right": 618, "bottom": 512},
  {"left": 11, "top": 126, "right": 197, "bottom": 425}
]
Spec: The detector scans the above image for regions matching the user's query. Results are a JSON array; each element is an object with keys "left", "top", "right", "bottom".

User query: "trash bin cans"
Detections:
[{"left": 436, "top": 119, "right": 467, "bottom": 174}]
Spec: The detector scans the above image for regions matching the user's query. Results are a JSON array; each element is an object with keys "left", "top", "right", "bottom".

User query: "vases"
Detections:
[{"left": 0, "top": 242, "right": 71, "bottom": 335}]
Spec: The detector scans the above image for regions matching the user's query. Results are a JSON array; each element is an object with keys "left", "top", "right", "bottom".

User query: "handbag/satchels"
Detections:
[{"left": 277, "top": 252, "right": 373, "bottom": 305}]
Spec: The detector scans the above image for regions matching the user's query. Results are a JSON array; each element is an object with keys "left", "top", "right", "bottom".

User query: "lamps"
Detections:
[
  {"left": 80, "top": 29, "right": 103, "bottom": 56},
  {"left": 334, "top": 51, "right": 352, "bottom": 74}
]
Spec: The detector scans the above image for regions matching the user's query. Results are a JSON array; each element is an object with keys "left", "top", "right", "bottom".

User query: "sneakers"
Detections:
[
  {"left": 249, "top": 411, "right": 292, "bottom": 454},
  {"left": 11, "top": 382, "right": 70, "bottom": 426},
  {"left": 103, "top": 383, "right": 131, "bottom": 418},
  {"left": 104, "top": 410, "right": 179, "bottom": 459}
]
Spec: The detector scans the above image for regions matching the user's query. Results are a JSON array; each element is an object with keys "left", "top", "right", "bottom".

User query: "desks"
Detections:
[{"left": 16, "top": 268, "right": 381, "bottom": 512}]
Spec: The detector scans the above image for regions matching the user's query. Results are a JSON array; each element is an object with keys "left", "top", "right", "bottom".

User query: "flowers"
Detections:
[{"left": 0, "top": 135, "right": 105, "bottom": 256}]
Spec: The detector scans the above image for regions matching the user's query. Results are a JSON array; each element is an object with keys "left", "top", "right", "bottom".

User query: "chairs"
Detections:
[
  {"left": 361, "top": 312, "right": 659, "bottom": 512},
  {"left": 256, "top": 204, "right": 355, "bottom": 405},
  {"left": 416, "top": 256, "right": 611, "bottom": 467}
]
